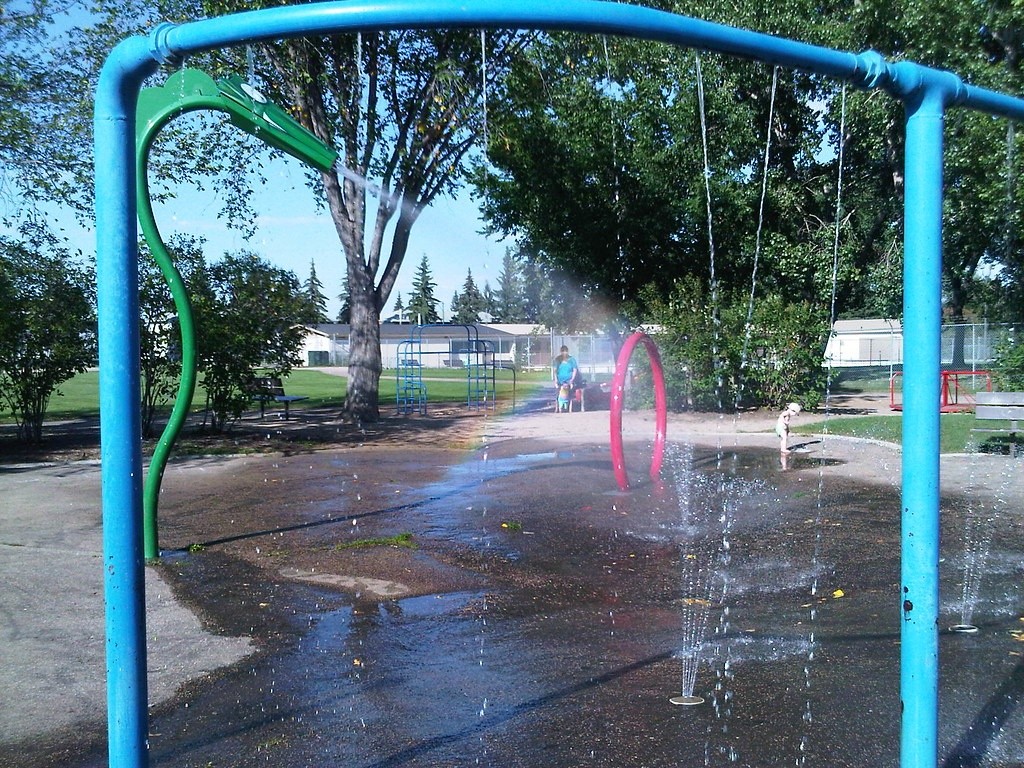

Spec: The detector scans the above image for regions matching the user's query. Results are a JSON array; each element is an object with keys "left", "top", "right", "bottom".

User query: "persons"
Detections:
[
  {"left": 556, "top": 382, "right": 574, "bottom": 412},
  {"left": 553, "top": 346, "right": 578, "bottom": 412},
  {"left": 776, "top": 403, "right": 801, "bottom": 451}
]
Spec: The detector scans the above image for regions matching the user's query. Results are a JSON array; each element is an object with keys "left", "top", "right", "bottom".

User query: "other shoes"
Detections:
[{"left": 781, "top": 450, "right": 791, "bottom": 456}]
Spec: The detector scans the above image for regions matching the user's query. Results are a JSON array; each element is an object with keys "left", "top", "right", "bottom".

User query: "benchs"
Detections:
[
  {"left": 970, "top": 391, "right": 1024, "bottom": 460},
  {"left": 240, "top": 378, "right": 310, "bottom": 421}
]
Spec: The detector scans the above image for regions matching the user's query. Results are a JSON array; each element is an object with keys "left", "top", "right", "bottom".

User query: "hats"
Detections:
[{"left": 788, "top": 403, "right": 801, "bottom": 415}]
